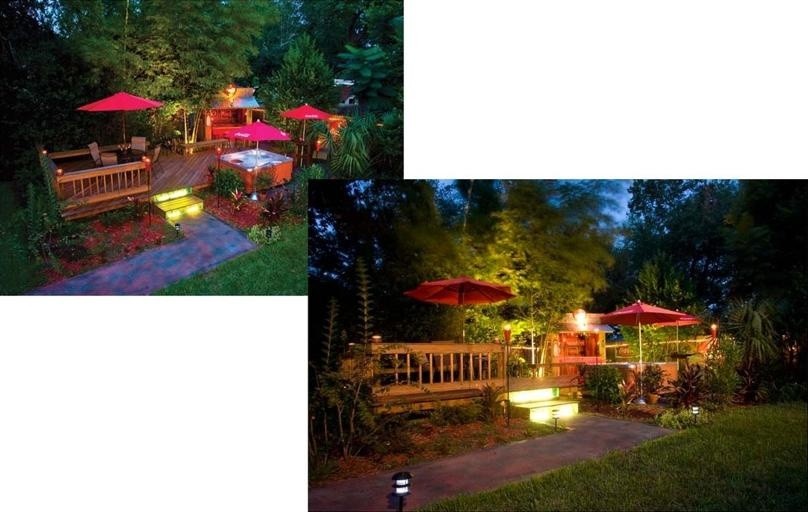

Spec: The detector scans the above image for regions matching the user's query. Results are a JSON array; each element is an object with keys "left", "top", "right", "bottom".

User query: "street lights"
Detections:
[
  {"left": 710, "top": 323, "right": 718, "bottom": 352},
  {"left": 144, "top": 158, "right": 152, "bottom": 216},
  {"left": 502, "top": 324, "right": 512, "bottom": 399},
  {"left": 315, "top": 140, "right": 321, "bottom": 166}
]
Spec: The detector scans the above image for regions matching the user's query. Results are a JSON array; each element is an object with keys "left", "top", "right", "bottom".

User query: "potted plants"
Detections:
[{"left": 642, "top": 363, "right": 673, "bottom": 404}]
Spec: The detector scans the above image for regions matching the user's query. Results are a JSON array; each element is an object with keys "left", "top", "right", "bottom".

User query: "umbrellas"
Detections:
[
  {"left": 600, "top": 298, "right": 689, "bottom": 361},
  {"left": 281, "top": 103, "right": 331, "bottom": 141},
  {"left": 403, "top": 273, "right": 518, "bottom": 343},
  {"left": 655, "top": 314, "right": 705, "bottom": 351},
  {"left": 76, "top": 90, "right": 164, "bottom": 139},
  {"left": 227, "top": 120, "right": 294, "bottom": 169}
]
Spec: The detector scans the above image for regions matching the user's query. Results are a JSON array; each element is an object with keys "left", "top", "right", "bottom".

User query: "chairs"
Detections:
[
  {"left": 87, "top": 135, "right": 165, "bottom": 186},
  {"left": 429, "top": 339, "right": 463, "bottom": 372}
]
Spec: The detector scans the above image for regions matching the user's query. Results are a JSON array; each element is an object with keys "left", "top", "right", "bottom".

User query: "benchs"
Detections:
[
  {"left": 180, "top": 138, "right": 229, "bottom": 158},
  {"left": 380, "top": 367, "right": 423, "bottom": 374}
]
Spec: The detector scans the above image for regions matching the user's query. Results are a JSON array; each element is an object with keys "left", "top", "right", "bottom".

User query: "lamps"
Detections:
[
  {"left": 573, "top": 307, "right": 587, "bottom": 321},
  {"left": 552, "top": 409, "right": 559, "bottom": 430},
  {"left": 175, "top": 224, "right": 180, "bottom": 237},
  {"left": 691, "top": 405, "right": 700, "bottom": 423}
]
